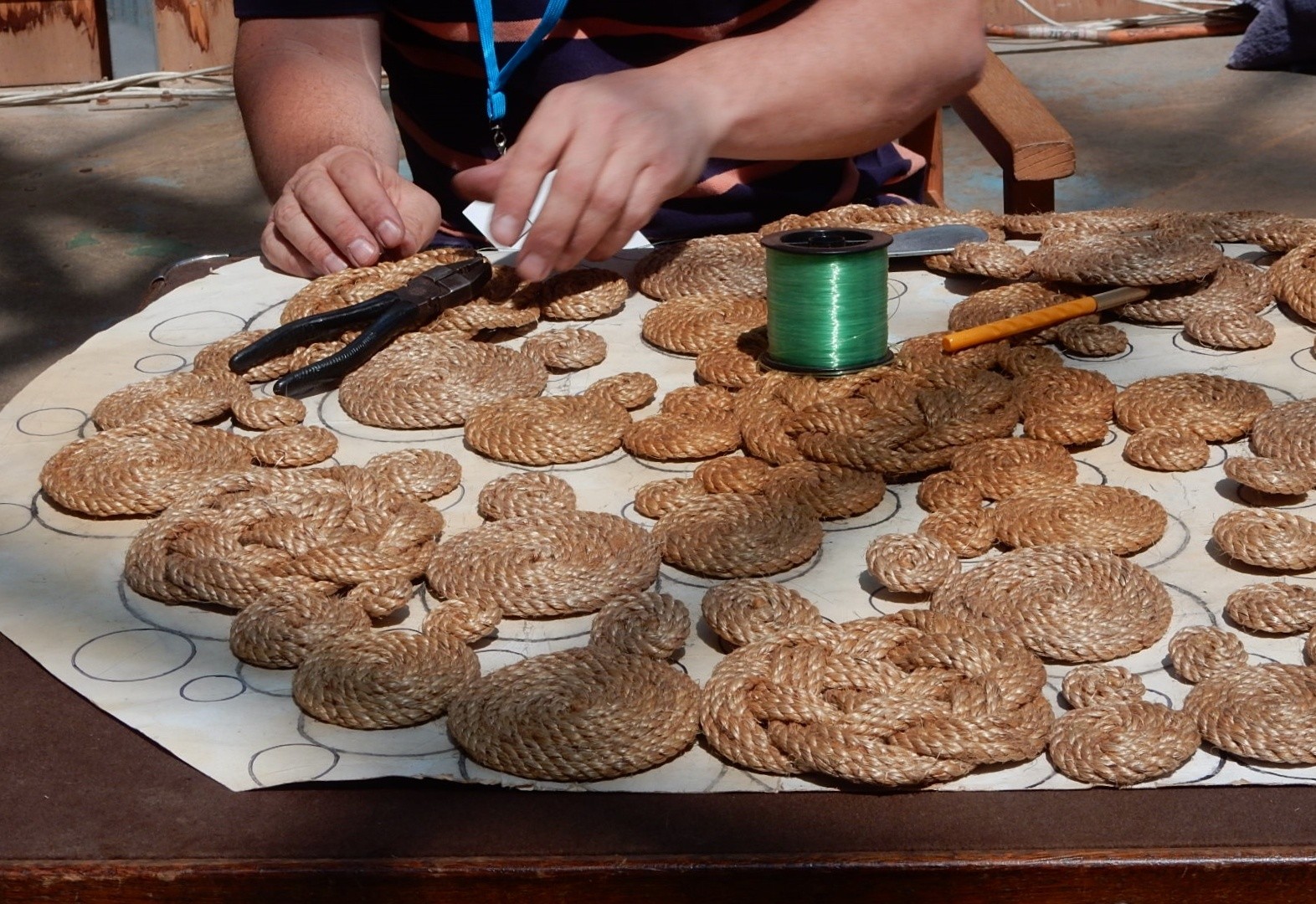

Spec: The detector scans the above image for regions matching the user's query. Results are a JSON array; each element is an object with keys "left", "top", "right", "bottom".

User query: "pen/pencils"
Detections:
[{"left": 941, "top": 285, "right": 1151, "bottom": 352}]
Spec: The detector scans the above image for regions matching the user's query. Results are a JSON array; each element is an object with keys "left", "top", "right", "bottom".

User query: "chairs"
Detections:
[{"left": 898, "top": 41, "right": 1076, "bottom": 212}]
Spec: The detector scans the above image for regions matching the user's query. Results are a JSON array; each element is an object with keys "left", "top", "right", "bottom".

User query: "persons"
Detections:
[{"left": 232, "top": 0, "right": 985, "bottom": 280}]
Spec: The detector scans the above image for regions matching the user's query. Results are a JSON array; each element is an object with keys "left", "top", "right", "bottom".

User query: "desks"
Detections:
[{"left": 0, "top": 214, "right": 1316, "bottom": 904}]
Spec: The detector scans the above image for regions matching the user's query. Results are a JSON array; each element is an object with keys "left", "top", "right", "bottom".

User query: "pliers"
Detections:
[{"left": 229, "top": 257, "right": 492, "bottom": 395}]
